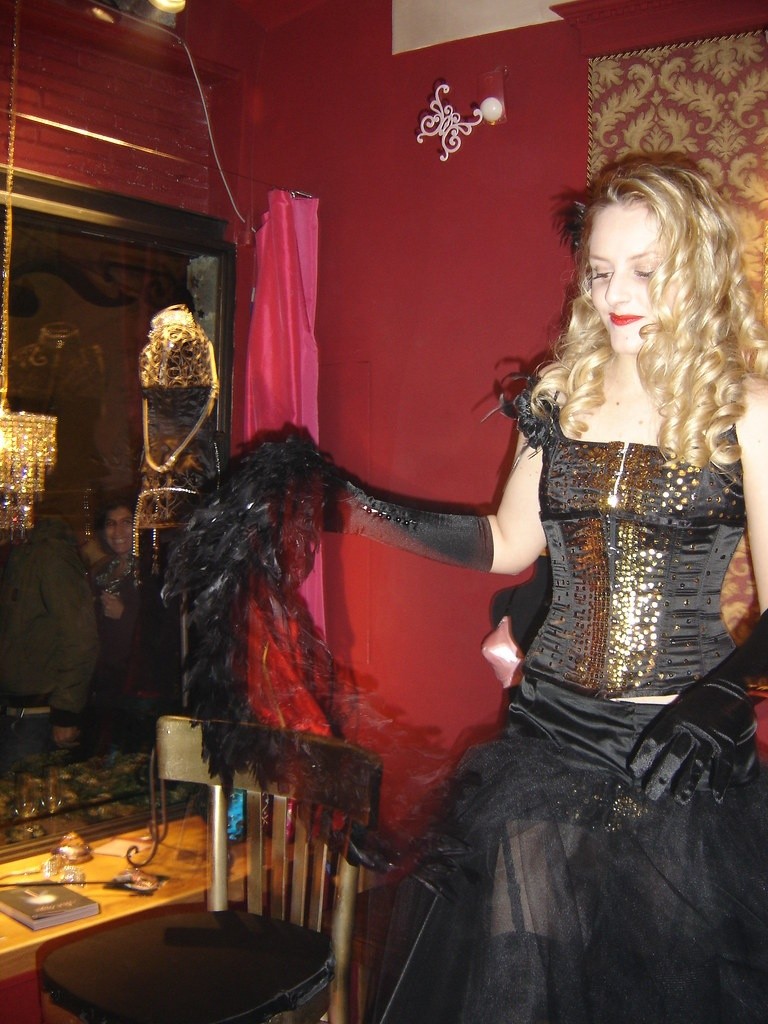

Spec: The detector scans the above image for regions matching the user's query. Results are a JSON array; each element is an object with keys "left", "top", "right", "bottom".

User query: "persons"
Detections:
[
  {"left": 276, "top": 164, "right": 768, "bottom": 1024},
  {"left": 0, "top": 497, "right": 180, "bottom": 785},
  {"left": 139, "top": 304, "right": 221, "bottom": 533}
]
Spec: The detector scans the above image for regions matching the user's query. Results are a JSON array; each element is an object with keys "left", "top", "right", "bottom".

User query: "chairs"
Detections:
[{"left": 37, "top": 712, "right": 385, "bottom": 1024}]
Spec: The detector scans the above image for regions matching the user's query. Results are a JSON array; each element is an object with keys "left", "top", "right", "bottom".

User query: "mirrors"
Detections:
[{"left": 0, "top": 164, "right": 233, "bottom": 863}]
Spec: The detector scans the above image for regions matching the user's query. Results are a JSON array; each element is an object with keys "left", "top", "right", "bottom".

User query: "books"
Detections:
[{"left": 0, "top": 879, "right": 100, "bottom": 931}]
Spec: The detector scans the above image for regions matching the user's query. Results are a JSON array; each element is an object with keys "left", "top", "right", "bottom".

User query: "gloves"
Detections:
[
  {"left": 628, "top": 610, "right": 768, "bottom": 808},
  {"left": 321, "top": 464, "right": 494, "bottom": 572}
]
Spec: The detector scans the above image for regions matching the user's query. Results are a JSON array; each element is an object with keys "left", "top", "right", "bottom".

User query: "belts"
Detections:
[{"left": 0, "top": 704, "right": 51, "bottom": 717}]
